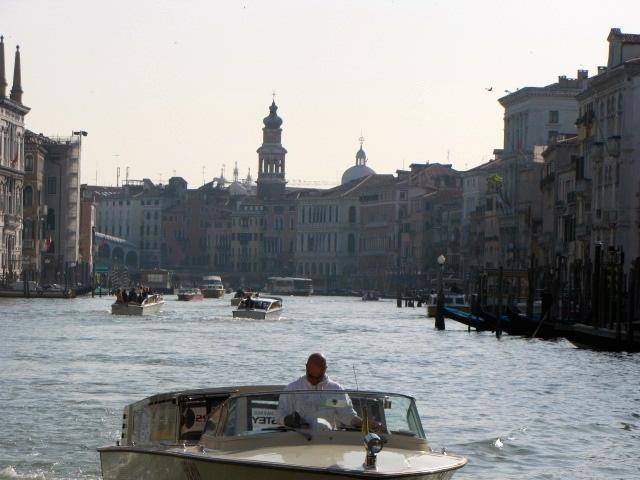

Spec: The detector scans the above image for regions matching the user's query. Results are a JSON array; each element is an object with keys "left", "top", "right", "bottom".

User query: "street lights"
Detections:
[{"left": 435, "top": 254, "right": 445, "bottom": 330}]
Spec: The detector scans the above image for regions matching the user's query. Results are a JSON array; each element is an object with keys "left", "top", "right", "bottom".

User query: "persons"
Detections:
[
  {"left": 275, "top": 352, "right": 386, "bottom": 434},
  {"left": 244, "top": 296, "right": 254, "bottom": 308},
  {"left": 255, "top": 294, "right": 262, "bottom": 306},
  {"left": 115, "top": 288, "right": 149, "bottom": 303}
]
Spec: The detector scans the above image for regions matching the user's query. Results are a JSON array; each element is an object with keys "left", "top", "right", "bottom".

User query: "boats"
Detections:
[
  {"left": 96, "top": 385, "right": 469, "bottom": 480},
  {"left": 231, "top": 276, "right": 313, "bottom": 320},
  {"left": 111, "top": 268, "right": 226, "bottom": 315}
]
